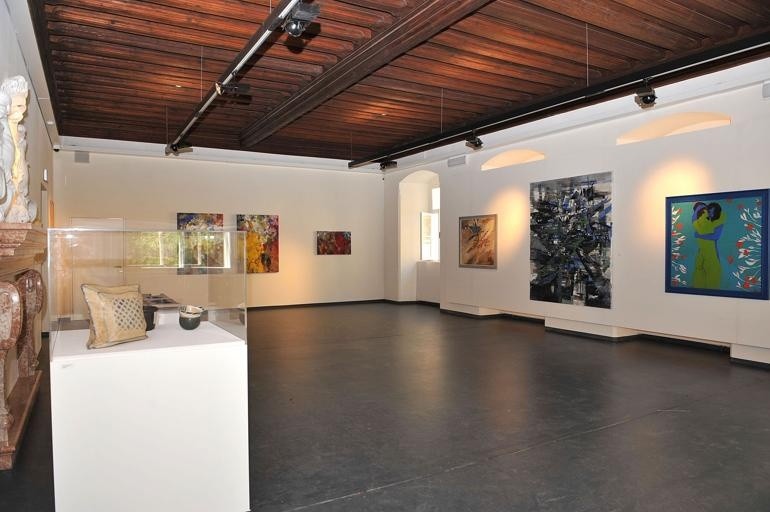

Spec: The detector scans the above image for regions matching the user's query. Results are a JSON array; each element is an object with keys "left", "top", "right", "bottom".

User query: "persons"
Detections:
[{"left": 0, "top": 76, "right": 39, "bottom": 224}]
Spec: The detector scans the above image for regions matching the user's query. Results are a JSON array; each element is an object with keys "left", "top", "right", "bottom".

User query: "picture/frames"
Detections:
[
  {"left": 663, "top": 187, "right": 769, "bottom": 301},
  {"left": 458, "top": 214, "right": 498, "bottom": 270}
]
